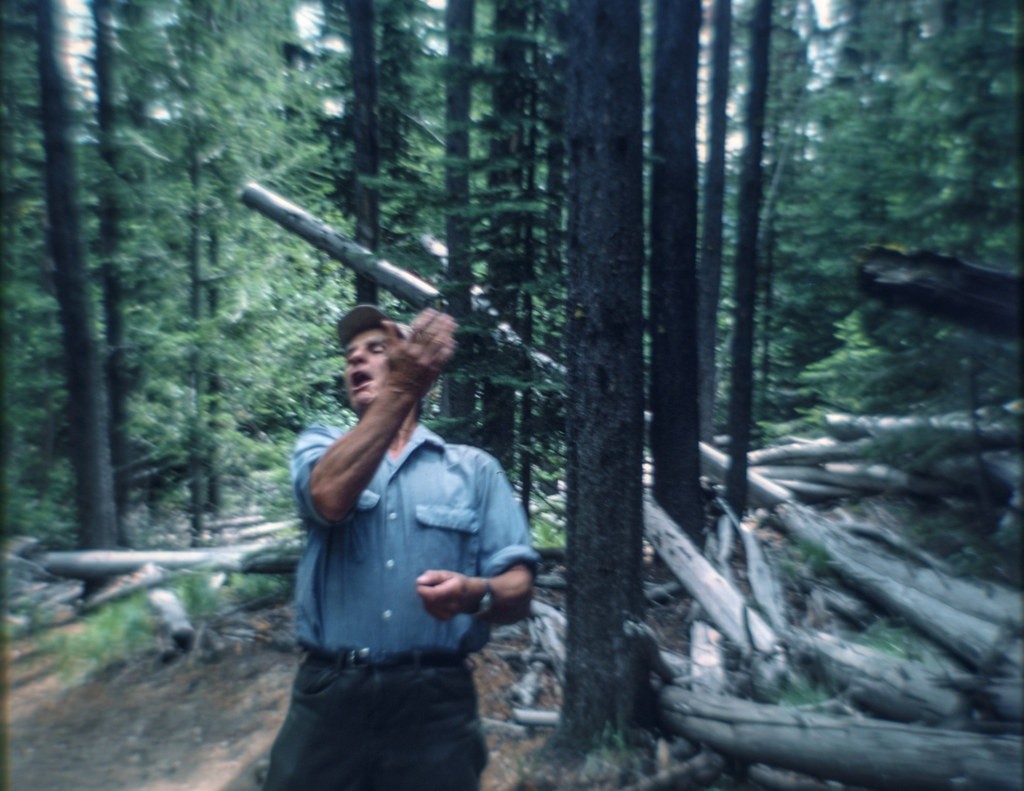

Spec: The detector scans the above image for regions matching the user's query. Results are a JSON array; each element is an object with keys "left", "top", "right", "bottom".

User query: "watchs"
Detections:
[{"left": 470, "top": 578, "right": 494, "bottom": 616}]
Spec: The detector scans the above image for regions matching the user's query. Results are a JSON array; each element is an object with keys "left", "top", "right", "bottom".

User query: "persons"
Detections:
[{"left": 261, "top": 305, "right": 540, "bottom": 790}]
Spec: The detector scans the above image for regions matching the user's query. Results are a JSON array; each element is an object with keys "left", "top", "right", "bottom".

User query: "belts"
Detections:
[{"left": 299, "top": 643, "right": 466, "bottom": 674}]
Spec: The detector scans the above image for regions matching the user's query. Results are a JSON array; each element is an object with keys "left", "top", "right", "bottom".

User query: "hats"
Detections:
[{"left": 335, "top": 303, "right": 414, "bottom": 342}]
